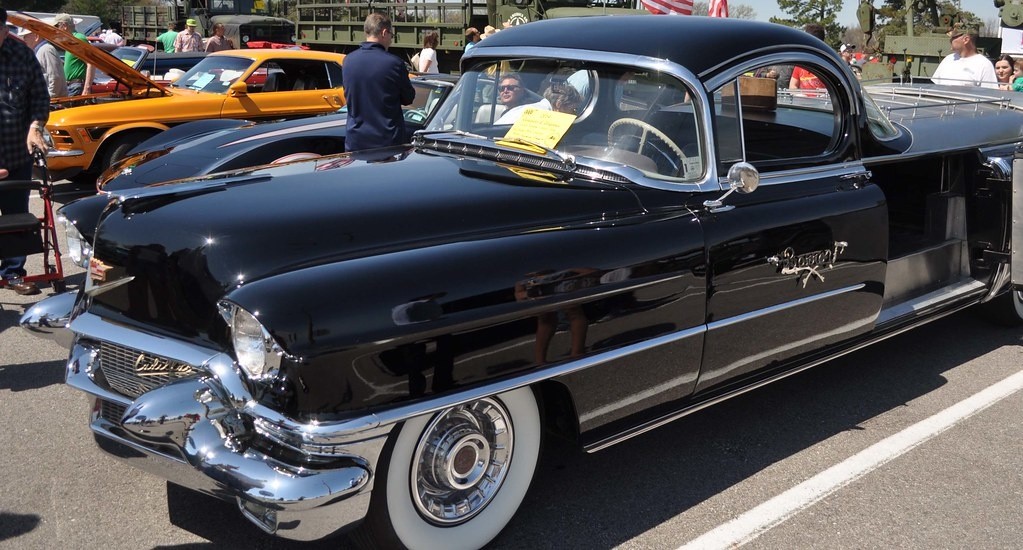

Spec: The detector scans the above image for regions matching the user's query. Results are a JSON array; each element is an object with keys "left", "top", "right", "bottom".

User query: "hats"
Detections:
[
  {"left": 17, "top": 26, "right": 32, "bottom": 36},
  {"left": 53, "top": 13, "right": 73, "bottom": 25},
  {"left": 946, "top": 23, "right": 979, "bottom": 37},
  {"left": 187, "top": 19, "right": 197, "bottom": 26},
  {"left": 168, "top": 21, "right": 176, "bottom": 26},
  {"left": 841, "top": 44, "right": 856, "bottom": 53},
  {"left": 480, "top": 25, "right": 500, "bottom": 40}
]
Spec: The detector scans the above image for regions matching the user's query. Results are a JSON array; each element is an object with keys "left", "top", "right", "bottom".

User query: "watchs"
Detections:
[{"left": 29, "top": 123, "right": 44, "bottom": 133}]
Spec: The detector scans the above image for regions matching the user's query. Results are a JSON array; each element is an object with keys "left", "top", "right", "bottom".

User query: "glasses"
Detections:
[
  {"left": 55, "top": 24, "right": 60, "bottom": 28},
  {"left": 845, "top": 50, "right": 853, "bottom": 53},
  {"left": 497, "top": 85, "right": 521, "bottom": 92},
  {"left": 387, "top": 30, "right": 397, "bottom": 39}
]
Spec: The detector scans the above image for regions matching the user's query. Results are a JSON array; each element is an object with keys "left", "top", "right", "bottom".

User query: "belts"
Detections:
[{"left": 71, "top": 79, "right": 82, "bottom": 83}]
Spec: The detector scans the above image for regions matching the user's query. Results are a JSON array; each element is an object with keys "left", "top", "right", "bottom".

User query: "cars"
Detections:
[
  {"left": 2, "top": 11, "right": 348, "bottom": 200},
  {"left": 18, "top": 9, "right": 1023, "bottom": 550}
]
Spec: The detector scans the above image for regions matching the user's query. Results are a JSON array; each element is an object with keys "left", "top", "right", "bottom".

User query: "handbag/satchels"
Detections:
[{"left": 411, "top": 52, "right": 420, "bottom": 71}]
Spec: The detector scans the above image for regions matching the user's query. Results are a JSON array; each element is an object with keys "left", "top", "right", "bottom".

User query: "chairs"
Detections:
[
  {"left": 293, "top": 69, "right": 320, "bottom": 90},
  {"left": 262, "top": 72, "right": 286, "bottom": 92}
]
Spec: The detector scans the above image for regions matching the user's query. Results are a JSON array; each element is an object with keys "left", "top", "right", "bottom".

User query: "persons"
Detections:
[
  {"left": 54, "top": 13, "right": 96, "bottom": 106},
  {"left": 418, "top": 32, "right": 440, "bottom": 75},
  {"left": 20, "top": 27, "right": 69, "bottom": 110},
  {"left": 839, "top": 43, "right": 882, "bottom": 76},
  {"left": 760, "top": 64, "right": 795, "bottom": 92},
  {"left": 479, "top": 71, "right": 585, "bottom": 131},
  {"left": 788, "top": 23, "right": 831, "bottom": 99},
  {"left": 464, "top": 21, "right": 527, "bottom": 82},
  {"left": 348, "top": 147, "right": 414, "bottom": 165},
  {"left": 124, "top": 243, "right": 229, "bottom": 348},
  {"left": 516, "top": 266, "right": 602, "bottom": 369},
  {"left": 342, "top": 12, "right": 416, "bottom": 151},
  {"left": 156, "top": 23, "right": 179, "bottom": 53},
  {"left": 175, "top": 18, "right": 203, "bottom": 53},
  {"left": 930, "top": 23, "right": 1000, "bottom": 90},
  {"left": 995, "top": 54, "right": 1023, "bottom": 91},
  {"left": 406, "top": 332, "right": 469, "bottom": 397},
  {"left": 87, "top": 24, "right": 126, "bottom": 53},
  {"left": 0, "top": 5, "right": 50, "bottom": 295},
  {"left": 203, "top": 22, "right": 233, "bottom": 52}
]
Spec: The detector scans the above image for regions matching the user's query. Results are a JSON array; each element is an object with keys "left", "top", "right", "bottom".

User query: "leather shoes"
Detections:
[{"left": 4, "top": 276, "right": 38, "bottom": 294}]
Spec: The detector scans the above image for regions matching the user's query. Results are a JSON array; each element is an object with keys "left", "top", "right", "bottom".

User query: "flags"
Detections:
[
  {"left": 708, "top": 0, "right": 728, "bottom": 19},
  {"left": 639, "top": 0, "right": 694, "bottom": 17}
]
